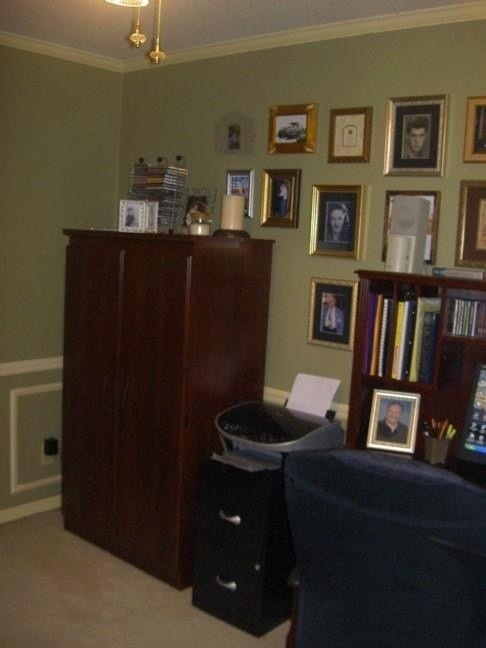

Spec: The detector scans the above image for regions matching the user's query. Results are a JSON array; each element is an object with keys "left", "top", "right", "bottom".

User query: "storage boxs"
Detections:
[
  {"left": 196, "top": 457, "right": 291, "bottom": 561},
  {"left": 192, "top": 544, "right": 289, "bottom": 637}
]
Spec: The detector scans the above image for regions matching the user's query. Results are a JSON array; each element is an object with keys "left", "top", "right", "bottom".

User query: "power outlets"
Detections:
[{"left": 41, "top": 445, "right": 56, "bottom": 465}]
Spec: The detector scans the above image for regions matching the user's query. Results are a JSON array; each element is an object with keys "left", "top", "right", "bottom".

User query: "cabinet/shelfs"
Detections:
[
  {"left": 62, "top": 229, "right": 275, "bottom": 590},
  {"left": 345, "top": 270, "right": 486, "bottom": 472}
]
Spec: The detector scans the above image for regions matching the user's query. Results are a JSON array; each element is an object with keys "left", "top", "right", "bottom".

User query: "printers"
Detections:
[{"left": 209, "top": 399, "right": 345, "bottom": 471}]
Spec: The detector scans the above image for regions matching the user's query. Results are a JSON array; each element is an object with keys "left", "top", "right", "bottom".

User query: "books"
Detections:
[
  {"left": 364, "top": 287, "right": 486, "bottom": 388},
  {"left": 129, "top": 163, "right": 189, "bottom": 225}
]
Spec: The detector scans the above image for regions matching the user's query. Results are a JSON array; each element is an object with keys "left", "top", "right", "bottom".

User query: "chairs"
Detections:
[{"left": 284, "top": 450, "right": 486, "bottom": 648}]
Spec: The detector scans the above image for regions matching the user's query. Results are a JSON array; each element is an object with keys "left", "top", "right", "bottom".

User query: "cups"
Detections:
[{"left": 422, "top": 437, "right": 449, "bottom": 466}]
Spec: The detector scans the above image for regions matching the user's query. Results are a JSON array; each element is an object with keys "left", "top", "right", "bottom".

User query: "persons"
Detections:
[
  {"left": 405, "top": 117, "right": 428, "bottom": 159},
  {"left": 126, "top": 208, "right": 138, "bottom": 227},
  {"left": 377, "top": 402, "right": 407, "bottom": 443},
  {"left": 323, "top": 295, "right": 343, "bottom": 334},
  {"left": 328, "top": 204, "right": 350, "bottom": 242},
  {"left": 274, "top": 180, "right": 290, "bottom": 216}
]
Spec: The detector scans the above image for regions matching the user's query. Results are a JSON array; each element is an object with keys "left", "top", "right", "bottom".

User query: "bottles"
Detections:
[{"left": 189, "top": 211, "right": 212, "bottom": 236}]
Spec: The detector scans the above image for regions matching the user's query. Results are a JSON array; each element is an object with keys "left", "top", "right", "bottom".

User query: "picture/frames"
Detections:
[
  {"left": 119, "top": 200, "right": 145, "bottom": 233},
  {"left": 455, "top": 180, "right": 486, "bottom": 268},
  {"left": 463, "top": 97, "right": 486, "bottom": 162},
  {"left": 146, "top": 202, "right": 158, "bottom": 233},
  {"left": 308, "top": 278, "right": 358, "bottom": 351},
  {"left": 382, "top": 190, "right": 440, "bottom": 265},
  {"left": 383, "top": 94, "right": 449, "bottom": 177},
  {"left": 367, "top": 390, "right": 421, "bottom": 454},
  {"left": 216, "top": 101, "right": 374, "bottom": 261}
]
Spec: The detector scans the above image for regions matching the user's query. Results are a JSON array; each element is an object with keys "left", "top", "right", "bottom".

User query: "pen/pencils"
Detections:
[{"left": 423, "top": 417, "right": 456, "bottom": 439}]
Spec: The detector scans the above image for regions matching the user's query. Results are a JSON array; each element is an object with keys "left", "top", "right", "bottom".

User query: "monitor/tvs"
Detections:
[{"left": 454, "top": 363, "right": 485, "bottom": 471}]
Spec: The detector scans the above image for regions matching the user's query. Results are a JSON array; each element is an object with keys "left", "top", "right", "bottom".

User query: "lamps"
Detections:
[{"left": 105, "top": 0, "right": 166, "bottom": 64}]
